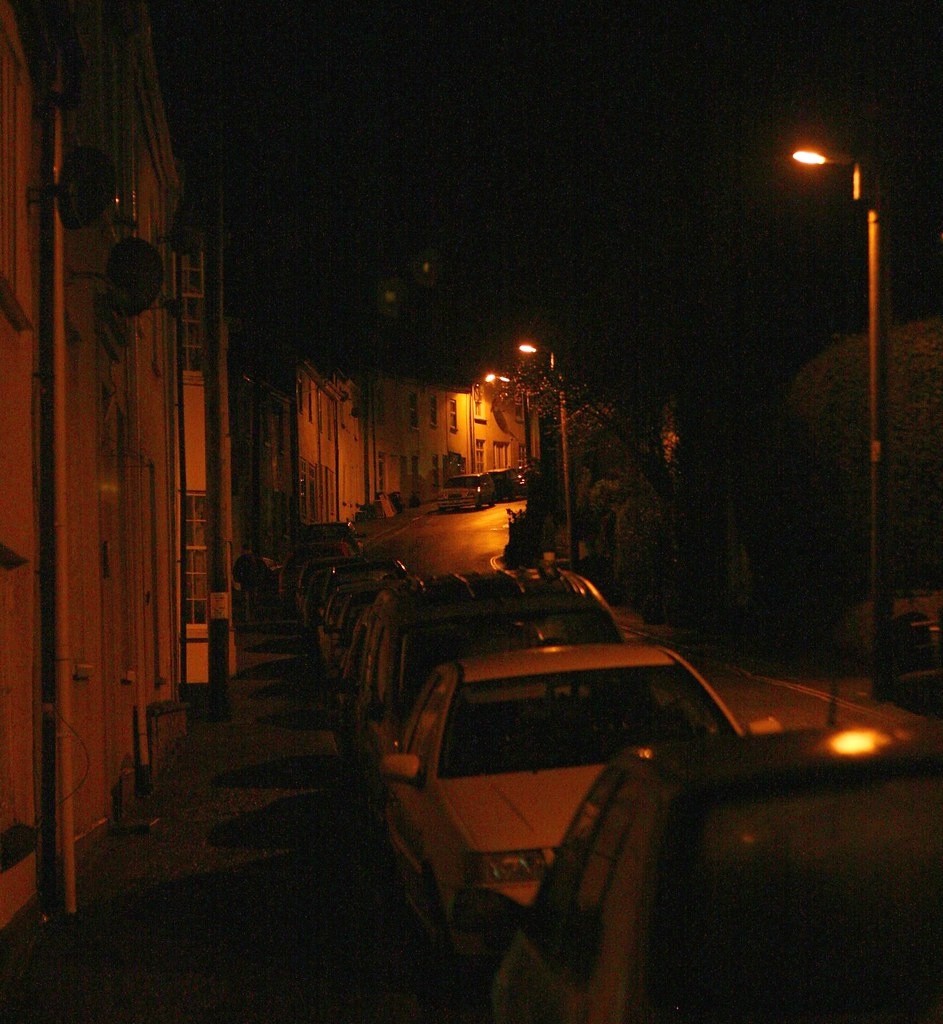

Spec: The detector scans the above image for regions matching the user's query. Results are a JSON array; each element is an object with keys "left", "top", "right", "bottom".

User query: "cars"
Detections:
[
  {"left": 278, "top": 519, "right": 625, "bottom": 823},
  {"left": 491, "top": 718, "right": 943, "bottom": 1024},
  {"left": 487, "top": 468, "right": 529, "bottom": 501},
  {"left": 437, "top": 473, "right": 496, "bottom": 512},
  {"left": 381, "top": 645, "right": 785, "bottom": 965}
]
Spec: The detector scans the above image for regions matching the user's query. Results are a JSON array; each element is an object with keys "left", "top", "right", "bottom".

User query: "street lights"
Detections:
[
  {"left": 519, "top": 344, "right": 573, "bottom": 571},
  {"left": 500, "top": 376, "right": 530, "bottom": 467},
  {"left": 471, "top": 373, "right": 495, "bottom": 472},
  {"left": 793, "top": 149, "right": 881, "bottom": 671}
]
